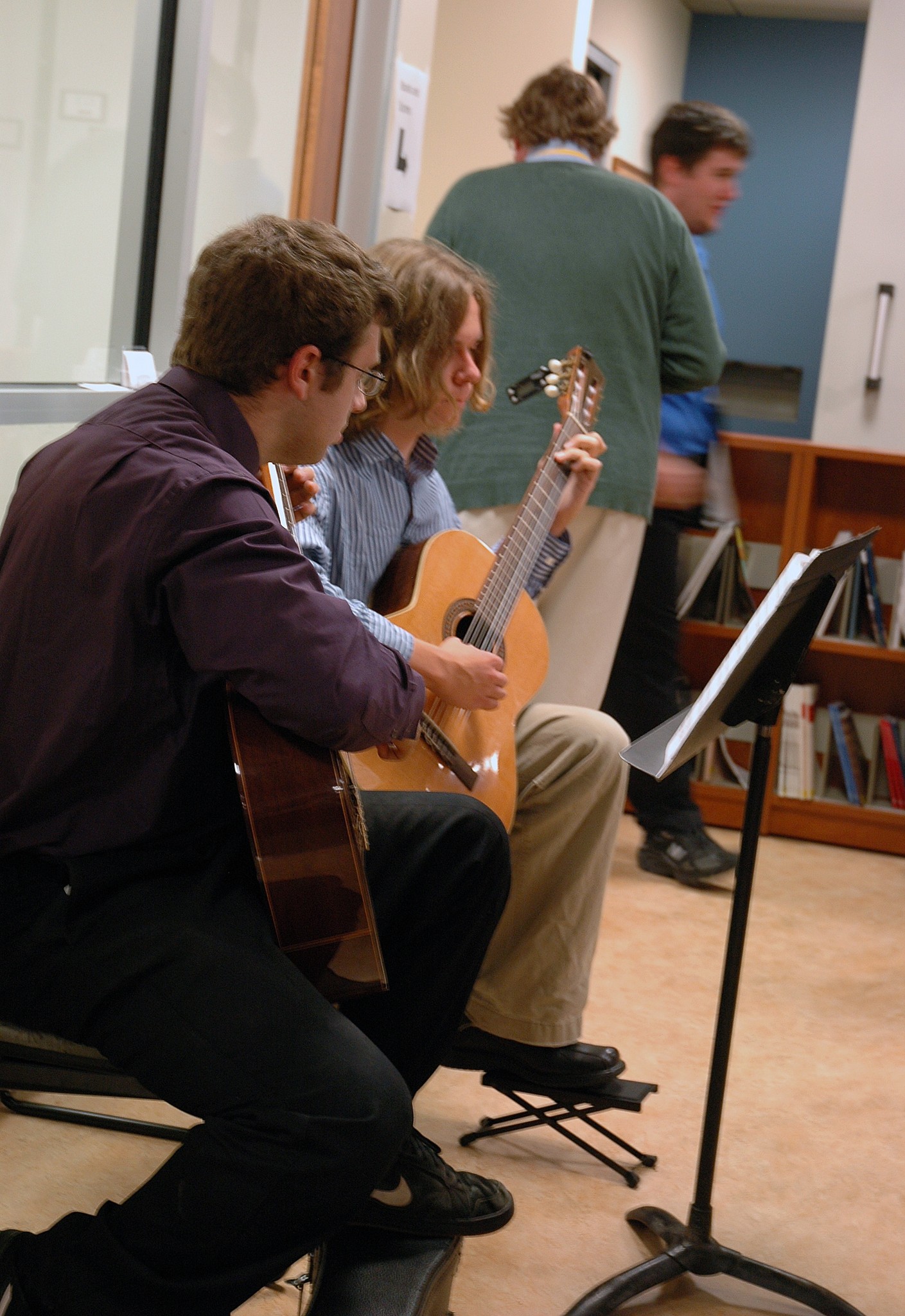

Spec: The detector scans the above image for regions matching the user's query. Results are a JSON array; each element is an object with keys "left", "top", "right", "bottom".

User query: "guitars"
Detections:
[
  {"left": 339, "top": 344, "right": 603, "bottom": 836},
  {"left": 227, "top": 462, "right": 390, "bottom": 1000}
]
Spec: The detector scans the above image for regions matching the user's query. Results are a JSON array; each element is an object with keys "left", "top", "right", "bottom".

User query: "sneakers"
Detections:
[
  {"left": 332, "top": 1129, "right": 517, "bottom": 1234},
  {"left": 0, "top": 1225, "right": 39, "bottom": 1316}
]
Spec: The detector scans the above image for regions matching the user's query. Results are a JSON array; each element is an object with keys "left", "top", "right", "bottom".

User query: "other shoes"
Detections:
[{"left": 639, "top": 824, "right": 740, "bottom": 884}]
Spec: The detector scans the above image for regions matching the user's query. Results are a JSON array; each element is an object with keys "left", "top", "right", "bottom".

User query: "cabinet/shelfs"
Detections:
[{"left": 626, "top": 428, "right": 905, "bottom": 861}]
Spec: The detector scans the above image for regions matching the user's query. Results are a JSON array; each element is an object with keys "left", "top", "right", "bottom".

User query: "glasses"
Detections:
[{"left": 323, "top": 353, "right": 392, "bottom": 398}]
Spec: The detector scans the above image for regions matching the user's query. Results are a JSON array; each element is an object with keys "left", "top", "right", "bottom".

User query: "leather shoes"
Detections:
[{"left": 441, "top": 1017, "right": 636, "bottom": 1089}]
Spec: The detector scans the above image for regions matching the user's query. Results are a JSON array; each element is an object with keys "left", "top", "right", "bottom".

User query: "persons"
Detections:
[
  {"left": 290, "top": 238, "right": 625, "bottom": 1089},
  {"left": 601, "top": 103, "right": 754, "bottom": 879},
  {"left": 0, "top": 214, "right": 516, "bottom": 1316},
  {"left": 421, "top": 65, "right": 727, "bottom": 711}
]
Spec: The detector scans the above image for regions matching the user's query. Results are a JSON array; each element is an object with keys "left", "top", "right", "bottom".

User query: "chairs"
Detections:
[{"left": 1, "top": 1014, "right": 202, "bottom": 1144}]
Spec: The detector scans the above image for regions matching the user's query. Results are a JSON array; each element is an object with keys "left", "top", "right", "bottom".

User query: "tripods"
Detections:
[{"left": 563, "top": 526, "right": 882, "bottom": 1316}]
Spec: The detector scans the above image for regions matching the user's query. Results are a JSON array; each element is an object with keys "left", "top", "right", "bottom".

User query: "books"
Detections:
[
  {"left": 693, "top": 733, "right": 749, "bottom": 790},
  {"left": 776, "top": 683, "right": 905, "bottom": 811},
  {"left": 675, "top": 519, "right": 756, "bottom": 624},
  {"left": 813, "top": 531, "right": 888, "bottom": 648}
]
